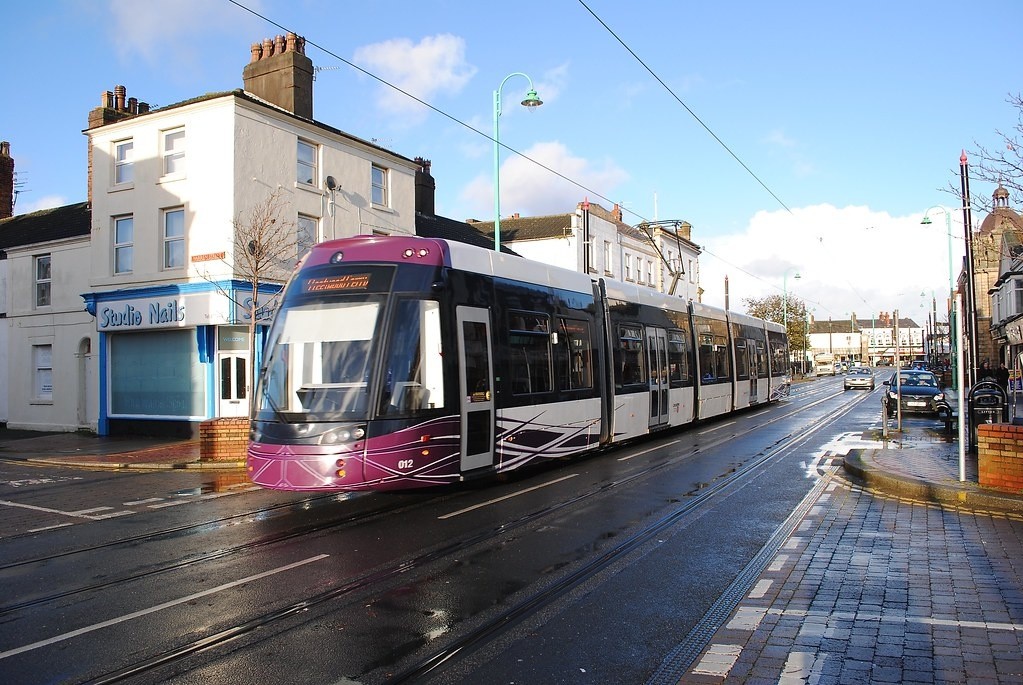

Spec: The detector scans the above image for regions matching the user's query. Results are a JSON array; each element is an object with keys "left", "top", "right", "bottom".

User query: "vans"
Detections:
[
  {"left": 911, "top": 361, "right": 927, "bottom": 371},
  {"left": 814, "top": 352, "right": 841, "bottom": 377}
]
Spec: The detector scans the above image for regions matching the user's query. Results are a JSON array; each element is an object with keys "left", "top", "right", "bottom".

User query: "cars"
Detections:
[
  {"left": 834, "top": 361, "right": 848, "bottom": 375},
  {"left": 882, "top": 369, "right": 945, "bottom": 418},
  {"left": 876, "top": 359, "right": 889, "bottom": 367},
  {"left": 841, "top": 366, "right": 876, "bottom": 390}
]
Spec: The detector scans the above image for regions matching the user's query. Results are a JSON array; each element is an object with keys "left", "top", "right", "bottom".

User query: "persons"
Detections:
[
  {"left": 944, "top": 358, "right": 952, "bottom": 370},
  {"left": 900, "top": 378, "right": 908, "bottom": 385},
  {"left": 917, "top": 379, "right": 930, "bottom": 386},
  {"left": 914, "top": 364, "right": 925, "bottom": 371},
  {"left": 980, "top": 362, "right": 992, "bottom": 381},
  {"left": 996, "top": 362, "right": 1010, "bottom": 403}
]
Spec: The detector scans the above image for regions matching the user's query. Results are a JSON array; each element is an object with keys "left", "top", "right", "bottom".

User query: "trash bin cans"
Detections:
[{"left": 968, "top": 381, "right": 1008, "bottom": 454}]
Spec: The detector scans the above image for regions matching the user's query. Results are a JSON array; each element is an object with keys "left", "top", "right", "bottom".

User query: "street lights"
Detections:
[
  {"left": 920, "top": 285, "right": 938, "bottom": 356},
  {"left": 493, "top": 70, "right": 545, "bottom": 252},
  {"left": 784, "top": 265, "right": 801, "bottom": 328},
  {"left": 920, "top": 205, "right": 957, "bottom": 392}
]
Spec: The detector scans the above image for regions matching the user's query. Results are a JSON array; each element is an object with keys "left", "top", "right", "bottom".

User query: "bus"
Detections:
[{"left": 246, "top": 220, "right": 790, "bottom": 495}]
